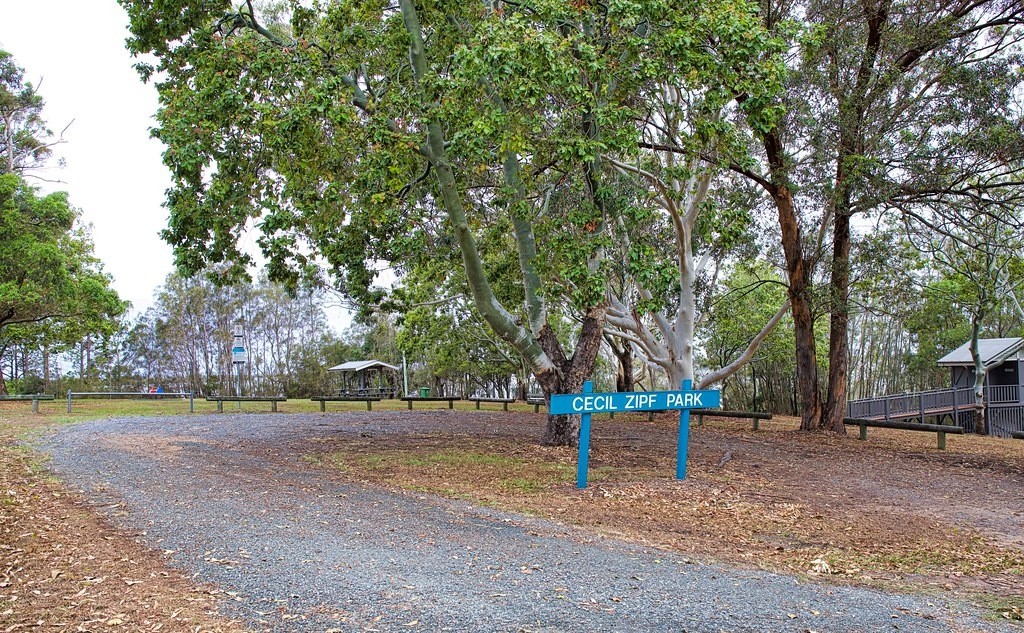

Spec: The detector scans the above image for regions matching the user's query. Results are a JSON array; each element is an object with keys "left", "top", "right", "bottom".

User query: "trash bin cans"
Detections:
[{"left": 419, "top": 386, "right": 431, "bottom": 398}]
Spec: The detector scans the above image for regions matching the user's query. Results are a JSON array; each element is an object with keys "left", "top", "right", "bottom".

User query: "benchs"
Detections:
[
  {"left": 843, "top": 417, "right": 964, "bottom": 449},
  {"left": 336, "top": 393, "right": 353, "bottom": 397},
  {"left": 358, "top": 393, "right": 388, "bottom": 397},
  {"left": 311, "top": 396, "right": 381, "bottom": 412},
  {"left": 527, "top": 400, "right": 546, "bottom": 413},
  {"left": 468, "top": 397, "right": 517, "bottom": 411},
  {"left": 400, "top": 397, "right": 461, "bottom": 410},
  {"left": 609, "top": 410, "right": 668, "bottom": 422},
  {"left": 690, "top": 409, "right": 773, "bottom": 430},
  {"left": 0, "top": 394, "right": 54, "bottom": 412},
  {"left": 206, "top": 396, "right": 287, "bottom": 413}
]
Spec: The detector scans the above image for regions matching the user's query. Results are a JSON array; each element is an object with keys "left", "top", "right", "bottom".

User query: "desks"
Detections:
[
  {"left": 334, "top": 389, "right": 359, "bottom": 394},
  {"left": 365, "top": 388, "right": 386, "bottom": 393}
]
